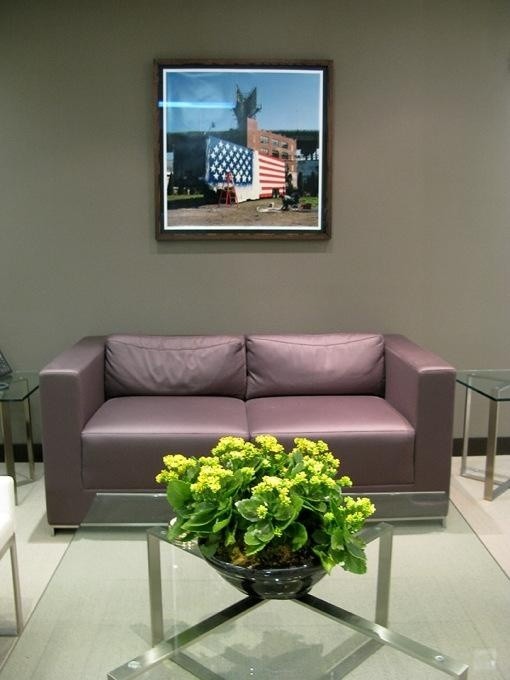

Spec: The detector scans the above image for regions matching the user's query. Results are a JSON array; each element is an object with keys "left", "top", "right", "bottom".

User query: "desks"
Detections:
[
  {"left": 454, "top": 370, "right": 509, "bottom": 502},
  {"left": 2, "top": 372, "right": 43, "bottom": 505}
]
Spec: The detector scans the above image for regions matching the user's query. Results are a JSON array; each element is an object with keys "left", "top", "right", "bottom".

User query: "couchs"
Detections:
[{"left": 38, "top": 330, "right": 455, "bottom": 530}]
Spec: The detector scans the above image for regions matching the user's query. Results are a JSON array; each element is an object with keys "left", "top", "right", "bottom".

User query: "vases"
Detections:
[{"left": 192, "top": 526, "right": 338, "bottom": 598}]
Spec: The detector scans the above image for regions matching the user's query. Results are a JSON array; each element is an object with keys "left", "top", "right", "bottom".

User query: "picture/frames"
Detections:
[{"left": 152, "top": 57, "right": 332, "bottom": 241}]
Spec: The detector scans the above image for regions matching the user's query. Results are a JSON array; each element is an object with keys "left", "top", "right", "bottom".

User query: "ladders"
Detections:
[{"left": 218, "top": 173, "right": 239, "bottom": 207}]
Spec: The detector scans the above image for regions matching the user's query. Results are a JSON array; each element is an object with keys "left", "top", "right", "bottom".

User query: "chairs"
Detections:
[{"left": 0, "top": 475, "right": 25, "bottom": 637}]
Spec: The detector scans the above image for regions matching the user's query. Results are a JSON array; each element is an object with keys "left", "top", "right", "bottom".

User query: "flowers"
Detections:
[{"left": 157, "top": 431, "right": 380, "bottom": 575}]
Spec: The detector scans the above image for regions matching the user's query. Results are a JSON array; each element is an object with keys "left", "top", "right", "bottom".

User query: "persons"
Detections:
[{"left": 279, "top": 194, "right": 297, "bottom": 211}]
